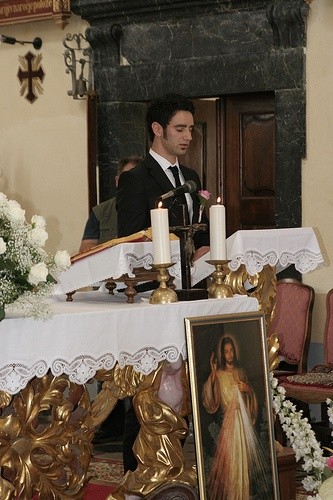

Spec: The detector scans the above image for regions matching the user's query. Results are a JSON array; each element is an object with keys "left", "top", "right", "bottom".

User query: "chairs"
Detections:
[{"left": 271, "top": 278, "right": 333, "bottom": 446}]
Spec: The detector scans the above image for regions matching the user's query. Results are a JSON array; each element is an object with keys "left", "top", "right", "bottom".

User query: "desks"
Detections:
[{"left": 0, "top": 290, "right": 262, "bottom": 500}]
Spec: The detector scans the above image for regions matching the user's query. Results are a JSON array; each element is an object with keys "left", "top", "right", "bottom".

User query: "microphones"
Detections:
[{"left": 160, "top": 180, "right": 197, "bottom": 200}]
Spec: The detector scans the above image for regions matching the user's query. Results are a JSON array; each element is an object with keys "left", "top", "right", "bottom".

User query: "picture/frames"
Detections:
[{"left": 184, "top": 310, "right": 278, "bottom": 500}]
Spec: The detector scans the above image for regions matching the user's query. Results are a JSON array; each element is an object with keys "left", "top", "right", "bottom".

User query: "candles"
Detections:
[
  {"left": 150, "top": 201, "right": 171, "bottom": 263},
  {"left": 209, "top": 197, "right": 227, "bottom": 260}
]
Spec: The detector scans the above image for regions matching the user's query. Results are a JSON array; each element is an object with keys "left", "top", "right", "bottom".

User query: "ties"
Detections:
[{"left": 167, "top": 165, "right": 190, "bottom": 224}]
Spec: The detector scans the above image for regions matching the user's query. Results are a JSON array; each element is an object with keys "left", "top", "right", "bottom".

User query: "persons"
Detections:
[
  {"left": 80, "top": 156, "right": 143, "bottom": 253},
  {"left": 115, "top": 93, "right": 211, "bottom": 476}
]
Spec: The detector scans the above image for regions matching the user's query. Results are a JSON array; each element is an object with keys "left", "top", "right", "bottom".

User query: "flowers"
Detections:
[
  {"left": 0, "top": 190, "right": 71, "bottom": 321},
  {"left": 198, "top": 189, "right": 211, "bottom": 221},
  {"left": 265, "top": 371, "right": 333, "bottom": 500}
]
